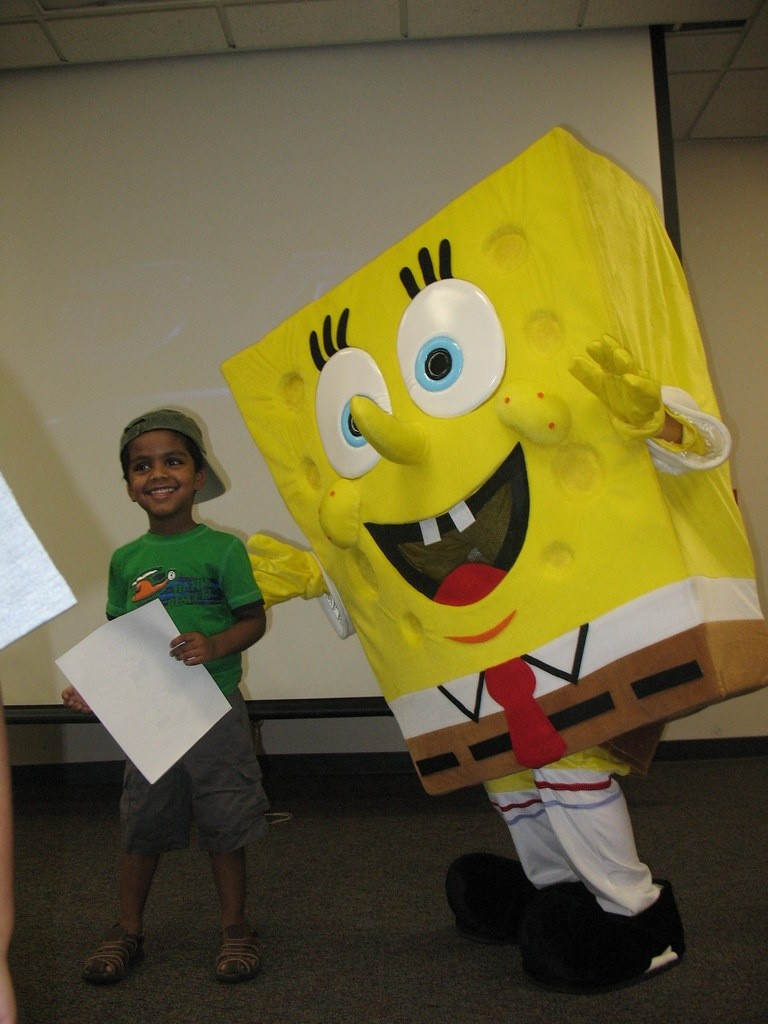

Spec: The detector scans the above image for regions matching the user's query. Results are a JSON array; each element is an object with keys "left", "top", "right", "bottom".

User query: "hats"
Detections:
[{"left": 119, "top": 407, "right": 227, "bottom": 504}]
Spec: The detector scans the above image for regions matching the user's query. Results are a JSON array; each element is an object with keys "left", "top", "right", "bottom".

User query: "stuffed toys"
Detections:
[{"left": 221, "top": 127, "right": 768, "bottom": 996}]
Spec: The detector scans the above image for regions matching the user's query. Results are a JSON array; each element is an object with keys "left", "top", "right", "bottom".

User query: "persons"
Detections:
[
  {"left": 61, "top": 410, "right": 267, "bottom": 985},
  {"left": 0, "top": 470, "right": 77, "bottom": 1024}
]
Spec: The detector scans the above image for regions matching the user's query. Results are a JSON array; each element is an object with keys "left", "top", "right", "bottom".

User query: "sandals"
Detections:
[
  {"left": 81, "top": 921, "right": 145, "bottom": 981},
  {"left": 215, "top": 918, "right": 269, "bottom": 983}
]
